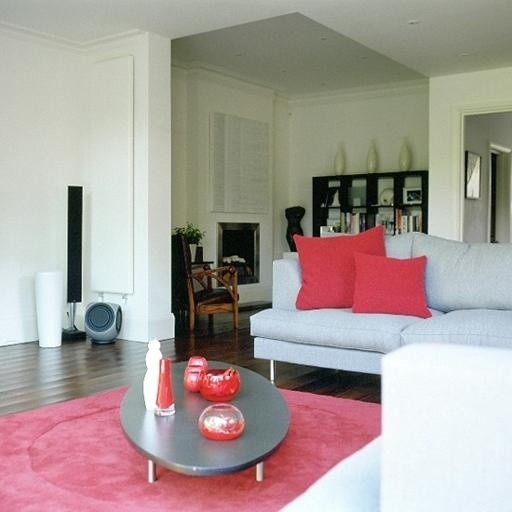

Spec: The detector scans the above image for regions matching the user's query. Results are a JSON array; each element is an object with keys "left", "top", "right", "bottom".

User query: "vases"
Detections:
[{"left": 366, "top": 142, "right": 378, "bottom": 173}]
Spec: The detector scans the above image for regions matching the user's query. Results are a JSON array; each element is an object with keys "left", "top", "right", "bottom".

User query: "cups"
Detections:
[{"left": 184, "top": 356, "right": 208, "bottom": 392}]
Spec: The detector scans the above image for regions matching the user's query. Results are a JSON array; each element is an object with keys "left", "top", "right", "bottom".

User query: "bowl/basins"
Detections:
[{"left": 199, "top": 369, "right": 240, "bottom": 402}]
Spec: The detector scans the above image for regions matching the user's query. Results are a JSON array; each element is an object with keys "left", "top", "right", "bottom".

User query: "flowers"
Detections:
[{"left": 223, "top": 255, "right": 246, "bottom": 267}]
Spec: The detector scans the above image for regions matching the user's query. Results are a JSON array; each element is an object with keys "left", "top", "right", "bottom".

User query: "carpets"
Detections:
[{"left": 0, "top": 385, "right": 381, "bottom": 512}]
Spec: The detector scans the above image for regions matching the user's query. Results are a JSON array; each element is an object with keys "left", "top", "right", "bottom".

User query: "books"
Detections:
[{"left": 318, "top": 207, "right": 422, "bottom": 236}]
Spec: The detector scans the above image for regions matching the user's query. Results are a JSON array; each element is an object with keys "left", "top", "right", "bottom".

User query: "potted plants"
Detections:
[{"left": 173, "top": 223, "right": 205, "bottom": 263}]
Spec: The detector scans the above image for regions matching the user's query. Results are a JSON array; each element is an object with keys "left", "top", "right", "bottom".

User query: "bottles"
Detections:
[
  {"left": 153, "top": 358, "right": 175, "bottom": 418},
  {"left": 198, "top": 404, "right": 245, "bottom": 441},
  {"left": 364, "top": 138, "right": 378, "bottom": 173}
]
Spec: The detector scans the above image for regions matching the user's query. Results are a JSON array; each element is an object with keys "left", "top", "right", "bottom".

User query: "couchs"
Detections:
[{"left": 248, "top": 250, "right": 511, "bottom": 388}]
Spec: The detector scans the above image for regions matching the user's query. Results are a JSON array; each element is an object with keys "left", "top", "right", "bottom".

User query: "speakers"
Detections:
[
  {"left": 84, "top": 301, "right": 123, "bottom": 345},
  {"left": 62, "top": 185, "right": 86, "bottom": 343}
]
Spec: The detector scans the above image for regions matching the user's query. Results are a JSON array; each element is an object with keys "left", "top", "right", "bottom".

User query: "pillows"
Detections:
[{"left": 293, "top": 225, "right": 433, "bottom": 318}]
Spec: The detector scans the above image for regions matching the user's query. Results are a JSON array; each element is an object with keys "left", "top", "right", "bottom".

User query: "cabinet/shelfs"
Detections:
[{"left": 312, "top": 170, "right": 430, "bottom": 237}]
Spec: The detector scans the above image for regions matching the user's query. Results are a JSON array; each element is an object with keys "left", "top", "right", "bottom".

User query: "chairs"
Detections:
[{"left": 172, "top": 233, "right": 240, "bottom": 330}]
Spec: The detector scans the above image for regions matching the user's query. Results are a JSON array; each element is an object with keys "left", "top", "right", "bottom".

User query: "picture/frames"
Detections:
[
  {"left": 403, "top": 187, "right": 422, "bottom": 205},
  {"left": 465, "top": 150, "right": 481, "bottom": 200}
]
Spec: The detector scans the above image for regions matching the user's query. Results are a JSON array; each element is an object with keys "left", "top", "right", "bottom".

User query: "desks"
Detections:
[{"left": 191, "top": 261, "right": 214, "bottom": 319}]
